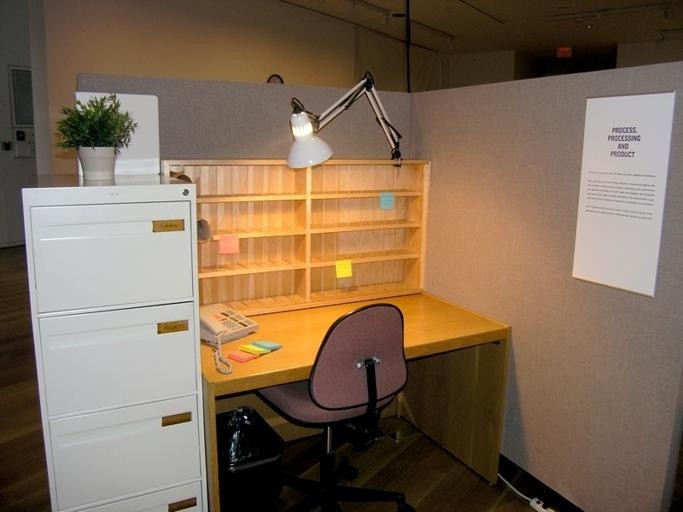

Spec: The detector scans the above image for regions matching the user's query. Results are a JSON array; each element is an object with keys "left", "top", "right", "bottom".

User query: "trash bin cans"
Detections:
[{"left": 216, "top": 406, "right": 287, "bottom": 511}]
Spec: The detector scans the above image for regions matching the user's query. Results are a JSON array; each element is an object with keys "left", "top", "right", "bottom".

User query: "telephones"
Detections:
[{"left": 199, "top": 303, "right": 259, "bottom": 345}]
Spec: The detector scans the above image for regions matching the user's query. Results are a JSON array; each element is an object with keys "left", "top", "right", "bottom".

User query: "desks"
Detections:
[{"left": 201, "top": 280, "right": 513, "bottom": 512}]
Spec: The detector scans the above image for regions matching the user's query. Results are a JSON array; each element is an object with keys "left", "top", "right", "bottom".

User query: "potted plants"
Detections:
[{"left": 53, "top": 86, "right": 140, "bottom": 182}]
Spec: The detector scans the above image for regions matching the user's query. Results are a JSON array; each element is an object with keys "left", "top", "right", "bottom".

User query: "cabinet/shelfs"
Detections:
[
  {"left": 21, "top": 174, "right": 210, "bottom": 512},
  {"left": 160, "top": 159, "right": 433, "bottom": 317}
]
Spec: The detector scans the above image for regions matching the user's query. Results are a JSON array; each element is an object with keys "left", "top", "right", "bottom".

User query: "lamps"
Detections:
[{"left": 286, "top": 70, "right": 404, "bottom": 168}]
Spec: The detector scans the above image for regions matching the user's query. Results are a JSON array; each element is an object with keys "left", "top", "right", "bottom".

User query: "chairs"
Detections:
[{"left": 253, "top": 302, "right": 411, "bottom": 512}]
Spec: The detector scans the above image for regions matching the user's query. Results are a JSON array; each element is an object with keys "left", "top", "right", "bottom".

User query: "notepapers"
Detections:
[{"left": 226, "top": 337, "right": 281, "bottom": 364}]
